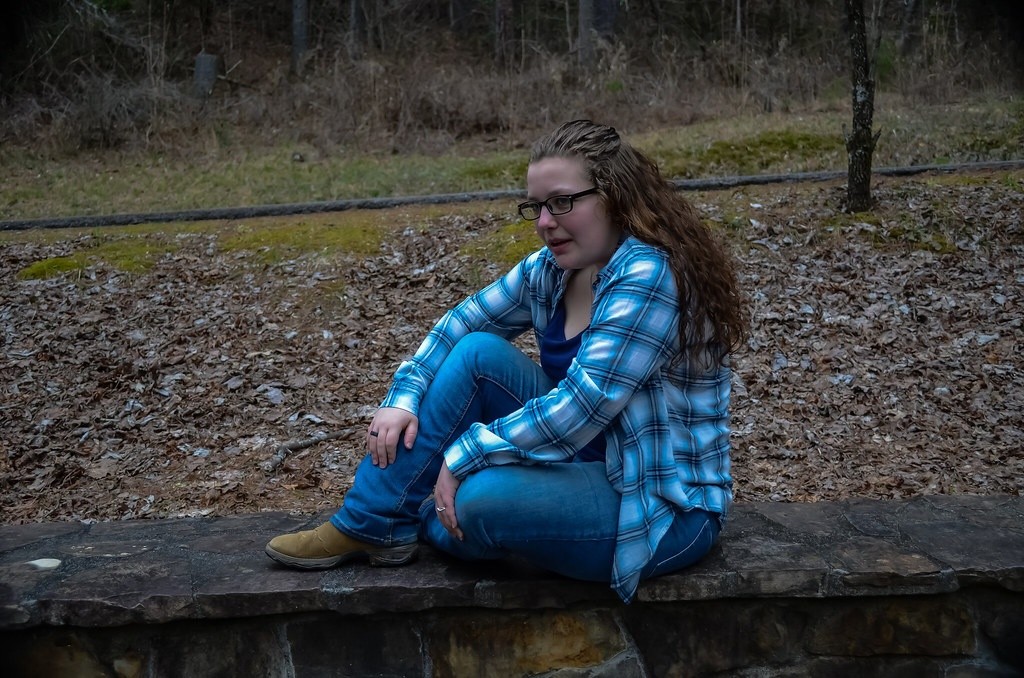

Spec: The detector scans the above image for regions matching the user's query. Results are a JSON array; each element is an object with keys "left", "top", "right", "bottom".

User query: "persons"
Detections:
[{"left": 265, "top": 115, "right": 754, "bottom": 609}]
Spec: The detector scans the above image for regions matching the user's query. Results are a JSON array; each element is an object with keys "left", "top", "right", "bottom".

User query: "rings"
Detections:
[
  {"left": 370, "top": 431, "right": 379, "bottom": 437},
  {"left": 436, "top": 506, "right": 446, "bottom": 512}
]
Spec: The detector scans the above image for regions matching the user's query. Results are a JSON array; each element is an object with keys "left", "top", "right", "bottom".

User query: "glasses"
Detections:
[{"left": 517, "top": 186, "right": 600, "bottom": 221}]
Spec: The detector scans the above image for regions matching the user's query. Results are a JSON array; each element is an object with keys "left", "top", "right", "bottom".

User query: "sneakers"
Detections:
[{"left": 264, "top": 520, "right": 418, "bottom": 567}]
